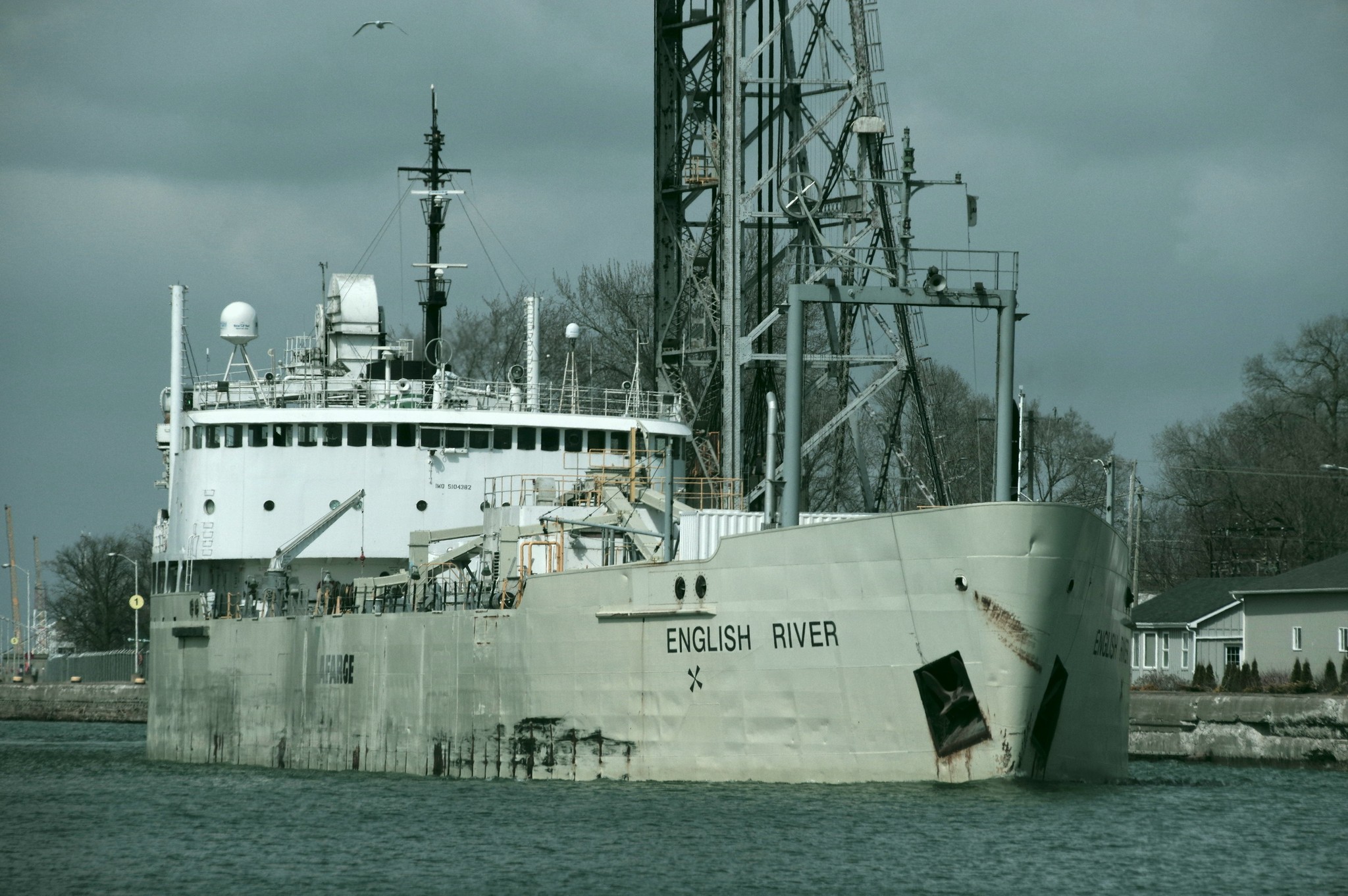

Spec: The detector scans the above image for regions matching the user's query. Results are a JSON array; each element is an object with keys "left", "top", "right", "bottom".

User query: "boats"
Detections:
[{"left": 140, "top": 82, "right": 1138, "bottom": 791}]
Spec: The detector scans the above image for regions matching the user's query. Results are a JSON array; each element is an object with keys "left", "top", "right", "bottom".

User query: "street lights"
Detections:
[
  {"left": 107, "top": 552, "right": 138, "bottom": 675},
  {"left": 2, "top": 563, "right": 31, "bottom": 674}
]
